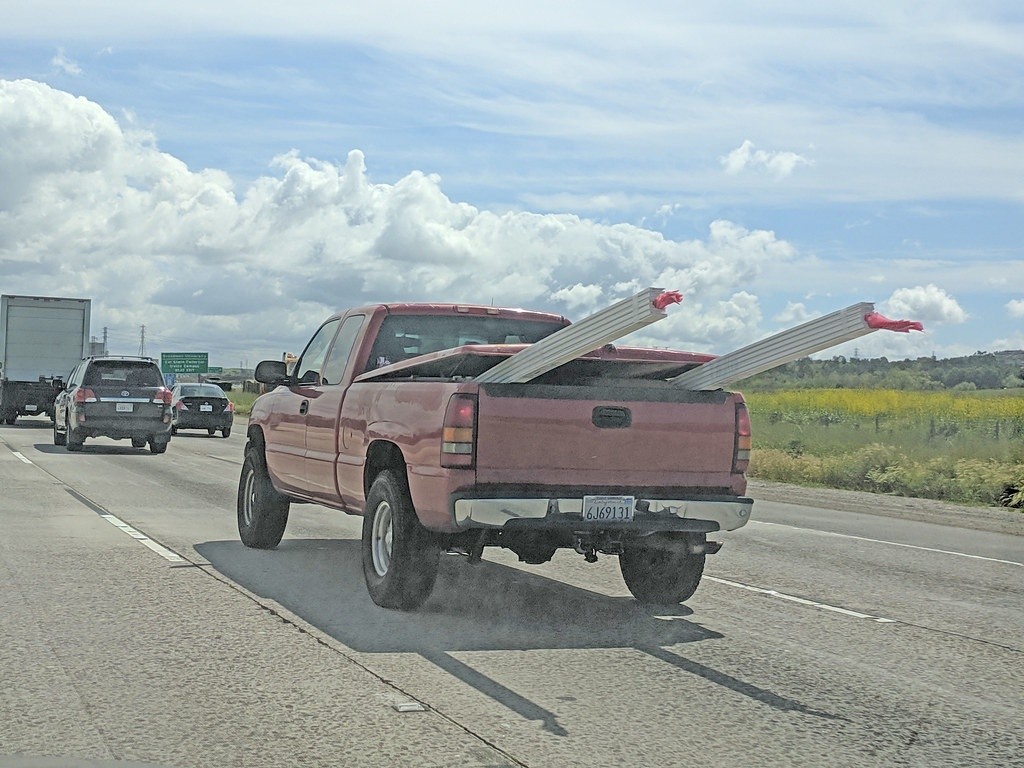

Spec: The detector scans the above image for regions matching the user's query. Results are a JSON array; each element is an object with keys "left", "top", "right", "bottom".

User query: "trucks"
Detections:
[{"left": 0, "top": 294, "right": 91, "bottom": 425}]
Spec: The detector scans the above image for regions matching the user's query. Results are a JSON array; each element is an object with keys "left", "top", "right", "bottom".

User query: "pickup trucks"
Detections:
[{"left": 237, "top": 303, "right": 754, "bottom": 609}]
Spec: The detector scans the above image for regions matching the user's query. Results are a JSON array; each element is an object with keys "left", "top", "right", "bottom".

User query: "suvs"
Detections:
[{"left": 54, "top": 354, "right": 173, "bottom": 453}]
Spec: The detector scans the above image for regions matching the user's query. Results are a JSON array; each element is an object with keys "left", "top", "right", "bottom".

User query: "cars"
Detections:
[{"left": 170, "top": 383, "right": 234, "bottom": 437}]
